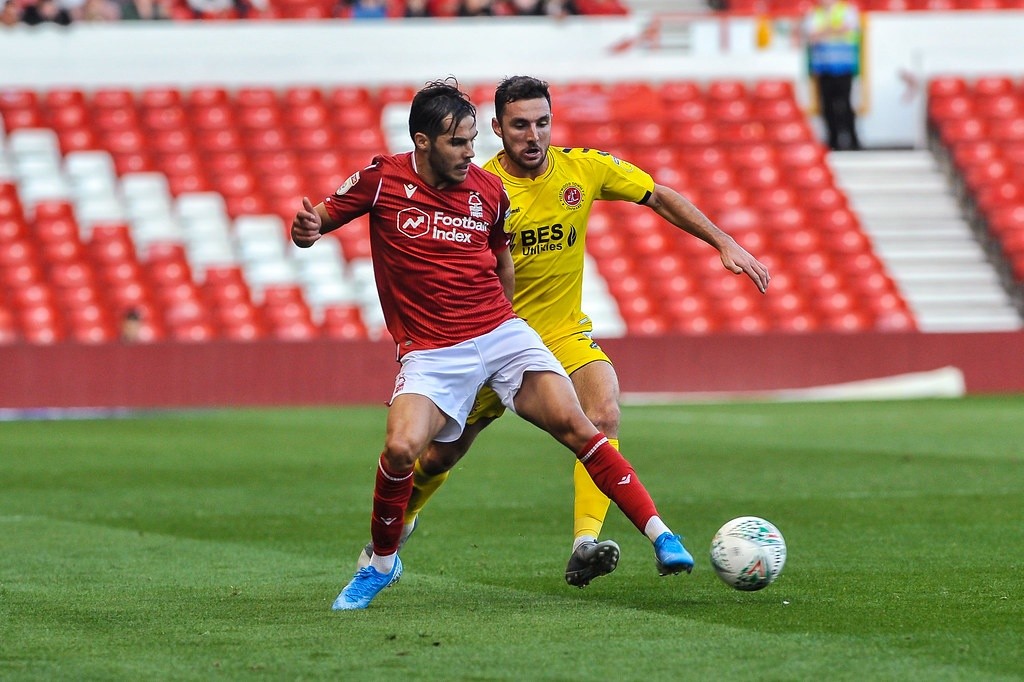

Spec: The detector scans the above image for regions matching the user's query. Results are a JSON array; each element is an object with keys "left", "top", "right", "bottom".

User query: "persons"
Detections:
[
  {"left": 0, "top": 0, "right": 582, "bottom": 25},
  {"left": 358, "top": 77, "right": 770, "bottom": 588},
  {"left": 293, "top": 77, "right": 694, "bottom": 610},
  {"left": 802, "top": 0, "right": 866, "bottom": 151}
]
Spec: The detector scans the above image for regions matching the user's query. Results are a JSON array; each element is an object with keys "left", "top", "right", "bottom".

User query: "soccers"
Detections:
[{"left": 710, "top": 516, "right": 786, "bottom": 591}]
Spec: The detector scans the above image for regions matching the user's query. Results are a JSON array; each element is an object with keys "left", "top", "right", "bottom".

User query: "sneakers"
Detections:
[
  {"left": 653, "top": 532, "right": 695, "bottom": 576},
  {"left": 330, "top": 553, "right": 403, "bottom": 609},
  {"left": 356, "top": 512, "right": 419, "bottom": 576},
  {"left": 564, "top": 540, "right": 621, "bottom": 590}
]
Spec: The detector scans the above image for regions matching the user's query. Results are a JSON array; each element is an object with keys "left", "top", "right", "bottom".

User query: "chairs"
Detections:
[
  {"left": 0, "top": 89, "right": 917, "bottom": 340},
  {"left": 928, "top": 76, "right": 1024, "bottom": 292}
]
